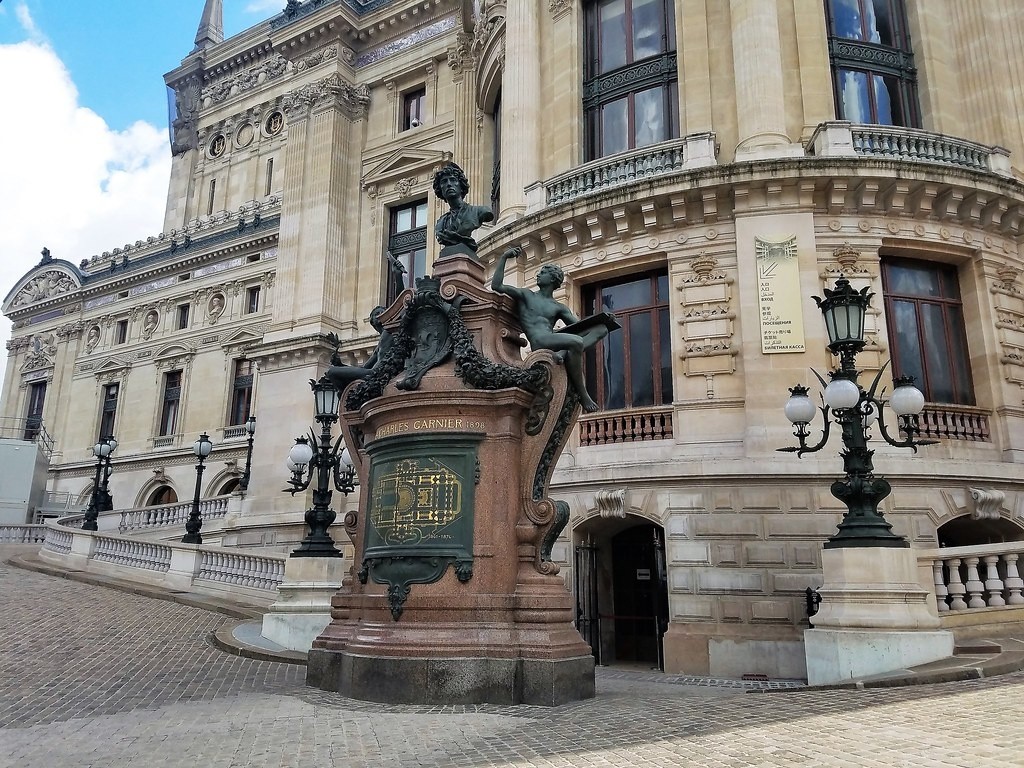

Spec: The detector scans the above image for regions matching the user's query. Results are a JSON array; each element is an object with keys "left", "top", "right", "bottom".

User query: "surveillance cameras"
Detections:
[{"left": 412, "top": 119, "right": 418, "bottom": 128}]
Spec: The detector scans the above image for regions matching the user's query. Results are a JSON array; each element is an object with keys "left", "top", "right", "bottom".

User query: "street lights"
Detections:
[
  {"left": 80, "top": 436, "right": 111, "bottom": 531},
  {"left": 237, "top": 415, "right": 256, "bottom": 492},
  {"left": 773, "top": 272, "right": 942, "bottom": 548},
  {"left": 282, "top": 370, "right": 359, "bottom": 557},
  {"left": 180, "top": 432, "right": 213, "bottom": 546},
  {"left": 97, "top": 433, "right": 118, "bottom": 511}
]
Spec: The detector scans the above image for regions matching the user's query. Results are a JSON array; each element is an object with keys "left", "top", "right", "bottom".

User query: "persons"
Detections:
[
  {"left": 431, "top": 166, "right": 494, "bottom": 252},
  {"left": 327, "top": 260, "right": 406, "bottom": 399},
  {"left": 491, "top": 246, "right": 617, "bottom": 412}
]
